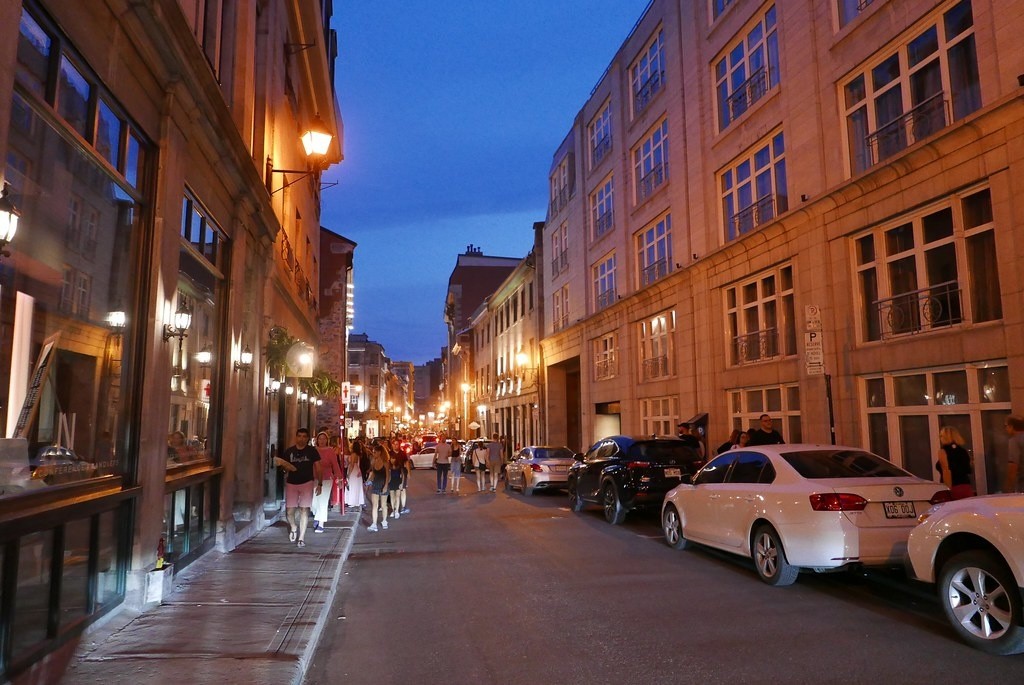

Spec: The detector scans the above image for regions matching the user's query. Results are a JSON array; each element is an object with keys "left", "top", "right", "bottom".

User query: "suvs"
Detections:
[{"left": 566, "top": 434, "right": 716, "bottom": 527}]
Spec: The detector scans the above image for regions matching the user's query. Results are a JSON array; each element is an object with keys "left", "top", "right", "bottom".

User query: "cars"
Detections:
[
  {"left": 659, "top": 442, "right": 954, "bottom": 587},
  {"left": 906, "top": 490, "right": 1024, "bottom": 657},
  {"left": 408, "top": 446, "right": 453, "bottom": 470},
  {"left": 502, "top": 445, "right": 580, "bottom": 497},
  {"left": 420, "top": 432, "right": 499, "bottom": 474}
]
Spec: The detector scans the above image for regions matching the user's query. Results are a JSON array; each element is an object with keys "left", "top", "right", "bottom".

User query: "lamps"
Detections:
[
  {"left": 198, "top": 341, "right": 212, "bottom": 379},
  {"left": 0, "top": 182, "right": 23, "bottom": 257},
  {"left": 162, "top": 298, "right": 193, "bottom": 351},
  {"left": 265, "top": 111, "right": 336, "bottom": 196},
  {"left": 234, "top": 341, "right": 253, "bottom": 379},
  {"left": 266, "top": 378, "right": 323, "bottom": 409},
  {"left": 106, "top": 299, "right": 127, "bottom": 346}
]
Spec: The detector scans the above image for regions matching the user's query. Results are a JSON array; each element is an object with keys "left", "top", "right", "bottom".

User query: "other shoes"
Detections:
[
  {"left": 381, "top": 521, "right": 389, "bottom": 529},
  {"left": 366, "top": 523, "right": 378, "bottom": 532},
  {"left": 436, "top": 486, "right": 497, "bottom": 495},
  {"left": 315, "top": 527, "right": 325, "bottom": 533},
  {"left": 395, "top": 511, "right": 400, "bottom": 519},
  {"left": 313, "top": 520, "right": 319, "bottom": 528},
  {"left": 390, "top": 512, "right": 396, "bottom": 518},
  {"left": 401, "top": 506, "right": 409, "bottom": 513}
]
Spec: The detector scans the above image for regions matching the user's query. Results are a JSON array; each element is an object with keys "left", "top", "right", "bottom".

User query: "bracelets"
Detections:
[{"left": 318, "top": 484, "right": 322, "bottom": 486}]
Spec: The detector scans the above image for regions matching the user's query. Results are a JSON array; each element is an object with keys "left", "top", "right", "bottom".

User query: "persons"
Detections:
[
  {"left": 473, "top": 441, "right": 490, "bottom": 491},
  {"left": 330, "top": 437, "right": 410, "bottom": 531},
  {"left": 935, "top": 426, "right": 973, "bottom": 499},
  {"left": 274, "top": 429, "right": 322, "bottom": 547},
  {"left": 676, "top": 422, "right": 706, "bottom": 458},
  {"left": 163, "top": 431, "right": 207, "bottom": 537},
  {"left": 389, "top": 428, "right": 449, "bottom": 454},
  {"left": 745, "top": 414, "right": 786, "bottom": 446},
  {"left": 486, "top": 434, "right": 504, "bottom": 492},
  {"left": 450, "top": 437, "right": 461, "bottom": 491},
  {"left": 311, "top": 432, "right": 341, "bottom": 532},
  {"left": 1005, "top": 415, "right": 1024, "bottom": 493},
  {"left": 95, "top": 432, "right": 114, "bottom": 476},
  {"left": 433, "top": 435, "right": 453, "bottom": 494},
  {"left": 717, "top": 428, "right": 756, "bottom": 454}
]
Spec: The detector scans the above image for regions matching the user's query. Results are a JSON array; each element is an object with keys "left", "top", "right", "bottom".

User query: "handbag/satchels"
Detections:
[{"left": 479, "top": 462, "right": 486, "bottom": 470}]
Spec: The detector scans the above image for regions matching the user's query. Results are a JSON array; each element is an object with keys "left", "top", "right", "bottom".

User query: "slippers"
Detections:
[
  {"left": 289, "top": 526, "right": 297, "bottom": 543},
  {"left": 297, "top": 540, "right": 305, "bottom": 548}
]
